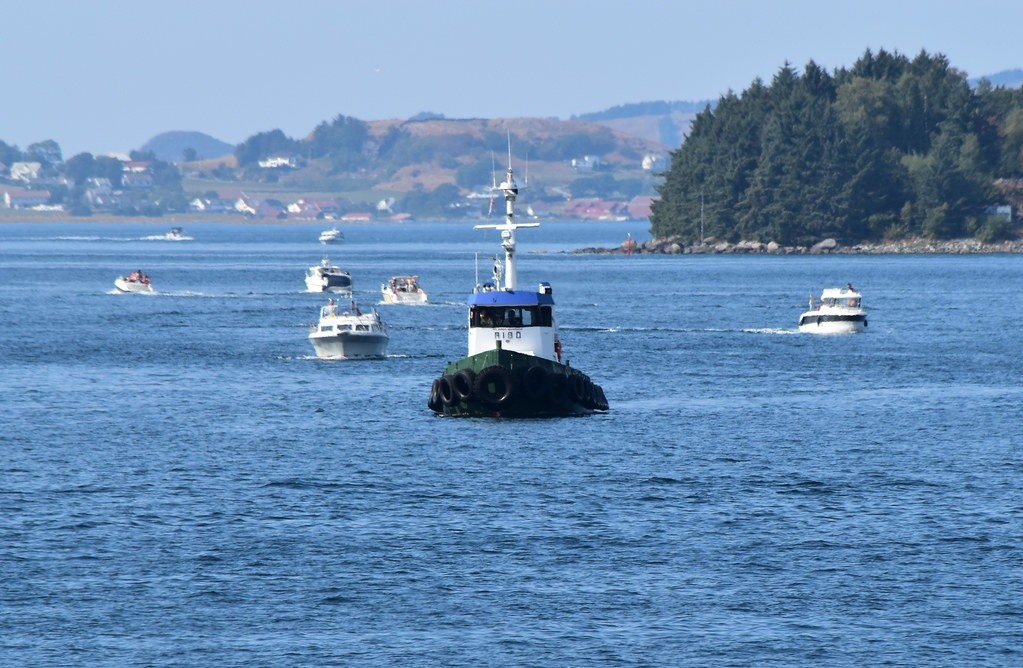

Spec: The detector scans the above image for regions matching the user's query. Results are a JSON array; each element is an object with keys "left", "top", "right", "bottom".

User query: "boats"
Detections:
[
  {"left": 319, "top": 231, "right": 344, "bottom": 244},
  {"left": 114, "top": 273, "right": 151, "bottom": 293},
  {"left": 168, "top": 227, "right": 184, "bottom": 240},
  {"left": 428, "top": 130, "right": 609, "bottom": 419},
  {"left": 380, "top": 275, "right": 427, "bottom": 305},
  {"left": 799, "top": 288, "right": 868, "bottom": 334},
  {"left": 307, "top": 297, "right": 389, "bottom": 360},
  {"left": 303, "top": 259, "right": 352, "bottom": 293}
]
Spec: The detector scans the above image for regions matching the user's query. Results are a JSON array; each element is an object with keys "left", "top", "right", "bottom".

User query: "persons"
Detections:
[
  {"left": 847, "top": 284, "right": 853, "bottom": 291},
  {"left": 480, "top": 309, "right": 519, "bottom": 327},
  {"left": 400, "top": 279, "right": 418, "bottom": 292},
  {"left": 325, "top": 298, "right": 337, "bottom": 314}
]
